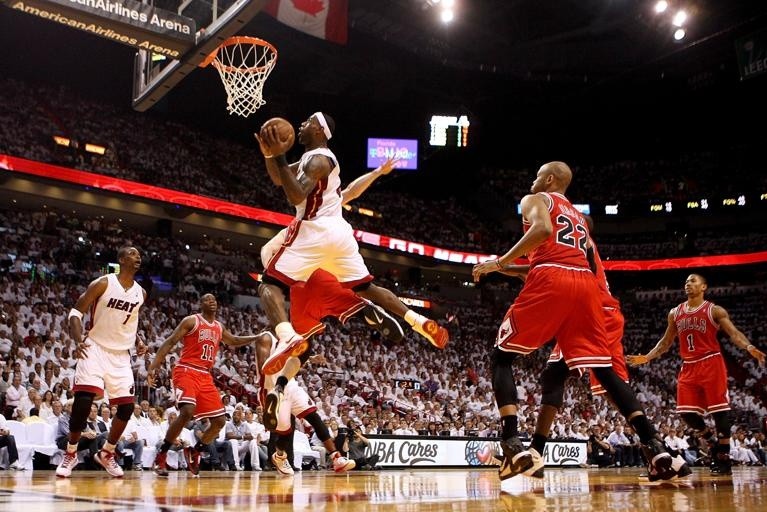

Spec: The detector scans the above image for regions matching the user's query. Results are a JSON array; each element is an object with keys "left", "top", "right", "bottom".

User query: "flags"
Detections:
[{"left": 264, "top": 0, "right": 349, "bottom": 42}]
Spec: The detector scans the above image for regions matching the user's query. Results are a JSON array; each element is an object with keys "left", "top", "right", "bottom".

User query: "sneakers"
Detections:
[
  {"left": 211, "top": 464, "right": 278, "bottom": 471},
  {"left": 642, "top": 433, "right": 694, "bottom": 481},
  {"left": 411, "top": 311, "right": 452, "bottom": 352},
  {"left": 149, "top": 452, "right": 173, "bottom": 479},
  {"left": 54, "top": 448, "right": 81, "bottom": 479},
  {"left": 259, "top": 332, "right": 310, "bottom": 376},
  {"left": 333, "top": 456, "right": 358, "bottom": 474},
  {"left": 588, "top": 463, "right": 643, "bottom": 469},
  {"left": 7, "top": 459, "right": 27, "bottom": 472},
  {"left": 706, "top": 448, "right": 735, "bottom": 478},
  {"left": 182, "top": 445, "right": 205, "bottom": 480},
  {"left": 92, "top": 448, "right": 126, "bottom": 479},
  {"left": 360, "top": 303, "right": 405, "bottom": 341},
  {"left": 130, "top": 463, "right": 145, "bottom": 472},
  {"left": 736, "top": 460, "right": 767, "bottom": 467},
  {"left": 493, "top": 435, "right": 547, "bottom": 483},
  {"left": 260, "top": 384, "right": 286, "bottom": 431},
  {"left": 271, "top": 449, "right": 296, "bottom": 477}
]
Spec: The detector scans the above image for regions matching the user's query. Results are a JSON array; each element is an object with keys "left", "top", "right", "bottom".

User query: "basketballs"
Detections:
[{"left": 260, "top": 118, "right": 295, "bottom": 149}]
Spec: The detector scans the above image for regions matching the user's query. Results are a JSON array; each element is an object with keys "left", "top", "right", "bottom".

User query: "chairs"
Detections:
[
  {"left": 292, "top": 430, "right": 319, "bottom": 470},
  {"left": 3, "top": 421, "right": 135, "bottom": 469}
]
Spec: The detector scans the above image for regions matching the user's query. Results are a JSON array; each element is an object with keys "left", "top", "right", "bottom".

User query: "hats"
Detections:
[{"left": 451, "top": 383, "right": 457, "bottom": 387}]
